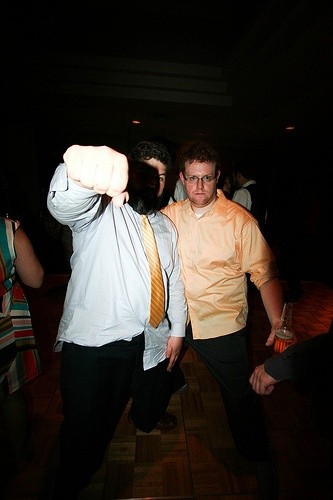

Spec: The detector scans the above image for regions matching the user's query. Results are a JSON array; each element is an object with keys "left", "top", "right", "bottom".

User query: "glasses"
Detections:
[{"left": 182, "top": 173, "right": 217, "bottom": 183}]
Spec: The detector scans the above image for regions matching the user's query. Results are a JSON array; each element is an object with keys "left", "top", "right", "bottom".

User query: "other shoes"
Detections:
[{"left": 155, "top": 411, "right": 178, "bottom": 431}]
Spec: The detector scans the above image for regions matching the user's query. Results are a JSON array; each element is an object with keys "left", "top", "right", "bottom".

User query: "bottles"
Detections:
[{"left": 272, "top": 302, "right": 295, "bottom": 356}]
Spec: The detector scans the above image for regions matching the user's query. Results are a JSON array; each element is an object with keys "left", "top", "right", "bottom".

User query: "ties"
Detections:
[{"left": 141, "top": 215, "right": 164, "bottom": 329}]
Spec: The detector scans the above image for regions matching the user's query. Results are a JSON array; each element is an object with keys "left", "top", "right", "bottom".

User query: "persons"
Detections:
[
  {"left": 168, "top": 169, "right": 276, "bottom": 239},
  {"left": 158, "top": 145, "right": 301, "bottom": 477},
  {"left": 249, "top": 327, "right": 333, "bottom": 395},
  {"left": 46, "top": 143, "right": 188, "bottom": 494},
  {"left": 0, "top": 207, "right": 48, "bottom": 440}
]
